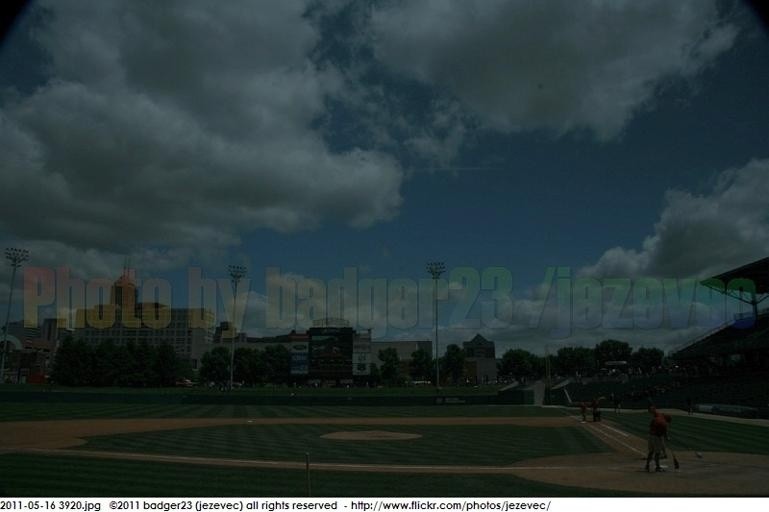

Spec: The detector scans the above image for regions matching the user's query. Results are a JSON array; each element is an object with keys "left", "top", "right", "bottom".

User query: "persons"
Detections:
[
  {"left": 645, "top": 406, "right": 666, "bottom": 472},
  {"left": 592, "top": 399, "right": 599, "bottom": 422},
  {"left": 580, "top": 402, "right": 588, "bottom": 423},
  {"left": 659, "top": 414, "right": 678, "bottom": 468}
]
